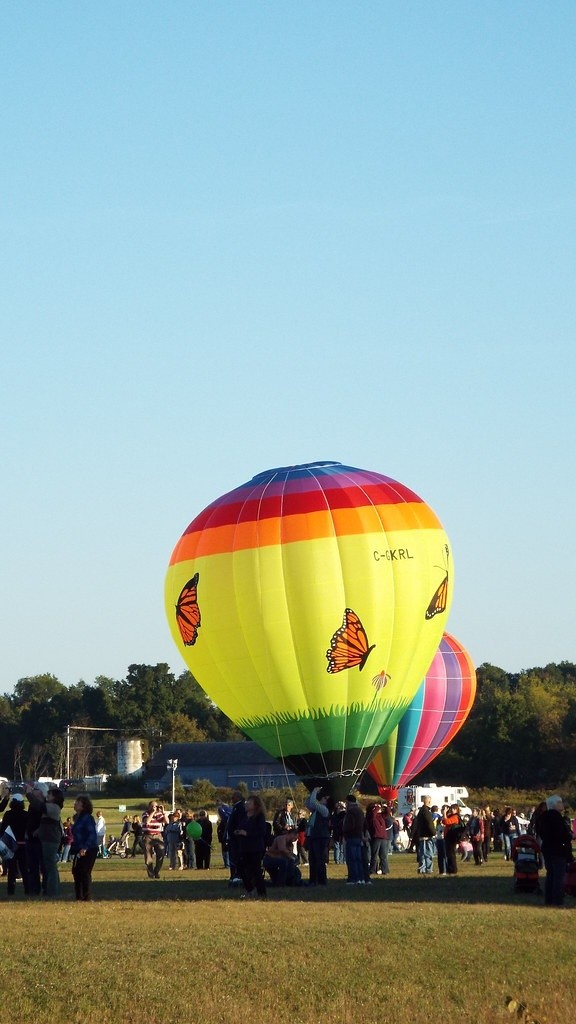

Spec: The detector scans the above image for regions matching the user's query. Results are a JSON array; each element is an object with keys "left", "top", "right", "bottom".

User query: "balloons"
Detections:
[{"left": 186, "top": 822, "right": 202, "bottom": 838}]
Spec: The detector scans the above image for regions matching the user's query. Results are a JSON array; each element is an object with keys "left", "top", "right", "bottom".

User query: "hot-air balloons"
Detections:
[
  {"left": 165, "top": 461, "right": 455, "bottom": 817},
  {"left": 360, "top": 632, "right": 476, "bottom": 817}
]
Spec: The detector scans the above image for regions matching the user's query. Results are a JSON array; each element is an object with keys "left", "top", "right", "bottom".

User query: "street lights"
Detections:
[{"left": 167, "top": 758, "right": 178, "bottom": 814}]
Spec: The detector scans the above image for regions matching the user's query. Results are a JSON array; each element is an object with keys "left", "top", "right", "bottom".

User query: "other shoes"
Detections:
[
  {"left": 479, "top": 859, "right": 484, "bottom": 864},
  {"left": 347, "top": 879, "right": 372, "bottom": 886},
  {"left": 377, "top": 869, "right": 387, "bottom": 875}
]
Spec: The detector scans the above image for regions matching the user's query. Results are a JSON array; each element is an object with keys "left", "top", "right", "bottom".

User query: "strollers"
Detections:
[
  {"left": 510, "top": 834, "right": 542, "bottom": 892},
  {"left": 106, "top": 831, "right": 132, "bottom": 859}
]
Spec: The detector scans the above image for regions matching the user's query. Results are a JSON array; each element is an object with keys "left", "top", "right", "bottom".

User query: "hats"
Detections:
[
  {"left": 9, "top": 793, "right": 25, "bottom": 802},
  {"left": 346, "top": 795, "right": 357, "bottom": 803}
]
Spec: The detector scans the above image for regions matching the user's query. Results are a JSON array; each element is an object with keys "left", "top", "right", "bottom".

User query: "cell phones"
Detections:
[{"left": 320, "top": 787, "right": 322, "bottom": 789}]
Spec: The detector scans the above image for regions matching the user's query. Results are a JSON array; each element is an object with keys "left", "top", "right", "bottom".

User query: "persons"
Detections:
[
  {"left": 264, "top": 799, "right": 309, "bottom": 882},
  {"left": 343, "top": 795, "right": 365, "bottom": 884},
  {"left": 403, "top": 796, "right": 576, "bottom": 875},
  {"left": 234, "top": 796, "right": 267, "bottom": 897},
  {"left": 72, "top": 796, "right": 98, "bottom": 901},
  {"left": 0, "top": 782, "right": 73, "bottom": 898},
  {"left": 107, "top": 791, "right": 247, "bottom": 887},
  {"left": 95, "top": 811, "right": 106, "bottom": 856},
  {"left": 306, "top": 787, "right": 335, "bottom": 886},
  {"left": 332, "top": 800, "right": 401, "bottom": 864},
  {"left": 366, "top": 801, "right": 394, "bottom": 875},
  {"left": 538, "top": 796, "right": 576, "bottom": 905}
]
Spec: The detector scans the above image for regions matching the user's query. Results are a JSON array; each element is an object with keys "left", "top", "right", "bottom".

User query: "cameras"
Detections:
[{"left": 156, "top": 806, "right": 161, "bottom": 811}]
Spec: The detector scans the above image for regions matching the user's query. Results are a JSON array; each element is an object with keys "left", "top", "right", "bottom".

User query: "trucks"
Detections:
[{"left": 397, "top": 785, "right": 473, "bottom": 821}]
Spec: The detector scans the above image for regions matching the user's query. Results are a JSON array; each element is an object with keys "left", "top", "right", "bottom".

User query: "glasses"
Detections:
[{"left": 199, "top": 813, "right": 206, "bottom": 816}]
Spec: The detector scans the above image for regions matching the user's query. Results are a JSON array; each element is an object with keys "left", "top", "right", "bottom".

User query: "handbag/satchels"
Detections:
[{"left": 449, "top": 812, "right": 466, "bottom": 843}]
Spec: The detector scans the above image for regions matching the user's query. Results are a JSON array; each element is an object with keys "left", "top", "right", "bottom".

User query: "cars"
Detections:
[{"left": 1, "top": 776, "right": 86, "bottom": 796}]
